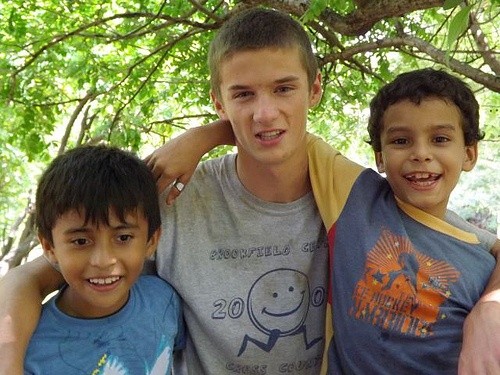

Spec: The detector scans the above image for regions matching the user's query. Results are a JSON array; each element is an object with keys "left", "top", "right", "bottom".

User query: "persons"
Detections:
[
  {"left": 0, "top": 9, "right": 500, "bottom": 375},
  {"left": 142, "top": 69, "right": 495, "bottom": 375},
  {"left": 24, "top": 144, "right": 186, "bottom": 375}
]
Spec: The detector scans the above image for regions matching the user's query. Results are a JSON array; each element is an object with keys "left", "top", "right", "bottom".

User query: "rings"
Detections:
[{"left": 175, "top": 180, "right": 185, "bottom": 192}]
